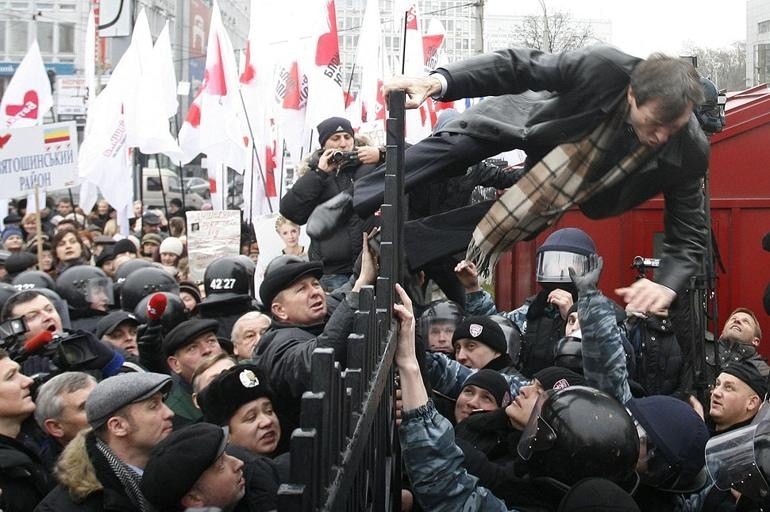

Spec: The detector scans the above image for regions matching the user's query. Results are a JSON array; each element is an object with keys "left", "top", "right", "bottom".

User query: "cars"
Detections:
[
  {"left": 142, "top": 167, "right": 244, "bottom": 211},
  {"left": 285, "top": 150, "right": 295, "bottom": 175}
]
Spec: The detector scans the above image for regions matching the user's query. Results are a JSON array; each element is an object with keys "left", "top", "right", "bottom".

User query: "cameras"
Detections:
[
  {"left": 632, "top": 256, "right": 662, "bottom": 277},
  {"left": 331, "top": 150, "right": 359, "bottom": 162}
]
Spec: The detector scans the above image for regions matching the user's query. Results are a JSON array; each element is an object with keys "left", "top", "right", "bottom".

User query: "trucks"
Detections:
[{"left": 54, "top": 74, "right": 91, "bottom": 128}]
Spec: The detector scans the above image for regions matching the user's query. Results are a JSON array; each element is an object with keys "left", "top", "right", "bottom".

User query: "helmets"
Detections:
[
  {"left": 533, "top": 228, "right": 600, "bottom": 284},
  {"left": 57, "top": 265, "right": 114, "bottom": 311},
  {"left": 420, "top": 300, "right": 460, "bottom": 352},
  {"left": 704, "top": 403, "right": 770, "bottom": 502},
  {"left": 123, "top": 267, "right": 179, "bottom": 308},
  {"left": 205, "top": 255, "right": 255, "bottom": 294},
  {"left": 0, "top": 269, "right": 54, "bottom": 320},
  {"left": 519, "top": 387, "right": 638, "bottom": 489},
  {"left": 625, "top": 392, "right": 706, "bottom": 489},
  {"left": 134, "top": 292, "right": 185, "bottom": 331}
]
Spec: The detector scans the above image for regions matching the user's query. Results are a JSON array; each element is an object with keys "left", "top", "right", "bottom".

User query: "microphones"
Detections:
[{"left": 145, "top": 293, "right": 167, "bottom": 326}]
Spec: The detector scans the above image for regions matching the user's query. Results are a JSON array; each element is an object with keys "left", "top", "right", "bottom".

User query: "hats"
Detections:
[
  {"left": 140, "top": 420, "right": 229, "bottom": 504},
  {"left": 0, "top": 196, "right": 187, "bottom": 269},
  {"left": 86, "top": 371, "right": 172, "bottom": 430},
  {"left": 179, "top": 281, "right": 200, "bottom": 303},
  {"left": 462, "top": 368, "right": 510, "bottom": 410},
  {"left": 198, "top": 362, "right": 267, "bottom": 425},
  {"left": 92, "top": 310, "right": 138, "bottom": 338},
  {"left": 162, "top": 317, "right": 220, "bottom": 354},
  {"left": 260, "top": 261, "right": 324, "bottom": 306},
  {"left": 533, "top": 365, "right": 588, "bottom": 394},
  {"left": 317, "top": 116, "right": 354, "bottom": 144},
  {"left": 722, "top": 361, "right": 766, "bottom": 403},
  {"left": 452, "top": 315, "right": 507, "bottom": 354}
]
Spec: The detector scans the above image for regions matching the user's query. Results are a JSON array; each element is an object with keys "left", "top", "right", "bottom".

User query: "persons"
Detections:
[
  {"left": 304, "top": 43, "right": 711, "bottom": 320},
  {"left": 405, "top": 108, "right": 525, "bottom": 310},
  {"left": 280, "top": 117, "right": 410, "bottom": 276}
]
